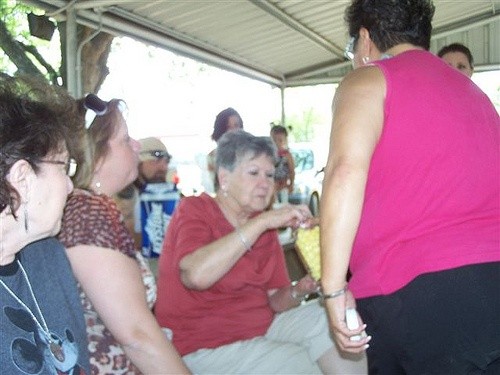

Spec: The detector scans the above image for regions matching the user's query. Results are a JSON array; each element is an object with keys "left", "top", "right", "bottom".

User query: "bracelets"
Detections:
[
  {"left": 316, "top": 282, "right": 349, "bottom": 298},
  {"left": 236, "top": 226, "right": 252, "bottom": 251},
  {"left": 291, "top": 280, "right": 309, "bottom": 300}
]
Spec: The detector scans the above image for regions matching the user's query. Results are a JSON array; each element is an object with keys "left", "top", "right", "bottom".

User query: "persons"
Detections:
[
  {"left": 0, "top": 91, "right": 92, "bottom": 375},
  {"left": 55, "top": 93, "right": 193, "bottom": 375},
  {"left": 153, "top": 129, "right": 368, "bottom": 375},
  {"left": 318, "top": 0, "right": 500, "bottom": 375},
  {"left": 114, "top": 108, "right": 295, "bottom": 244},
  {"left": 436, "top": 42, "right": 474, "bottom": 79}
]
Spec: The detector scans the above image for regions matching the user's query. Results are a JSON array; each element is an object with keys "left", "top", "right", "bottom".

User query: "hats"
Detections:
[{"left": 144, "top": 137, "right": 170, "bottom": 162}]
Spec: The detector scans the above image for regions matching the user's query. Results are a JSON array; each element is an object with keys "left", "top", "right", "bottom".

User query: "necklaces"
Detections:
[
  {"left": 95, "top": 173, "right": 104, "bottom": 195},
  {"left": 0, "top": 259, "right": 65, "bottom": 363}
]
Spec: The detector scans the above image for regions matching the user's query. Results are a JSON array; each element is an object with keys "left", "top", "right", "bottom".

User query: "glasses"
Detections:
[
  {"left": 340, "top": 36, "right": 367, "bottom": 62},
  {"left": 26, "top": 151, "right": 79, "bottom": 179},
  {"left": 72, "top": 92, "right": 110, "bottom": 129}
]
